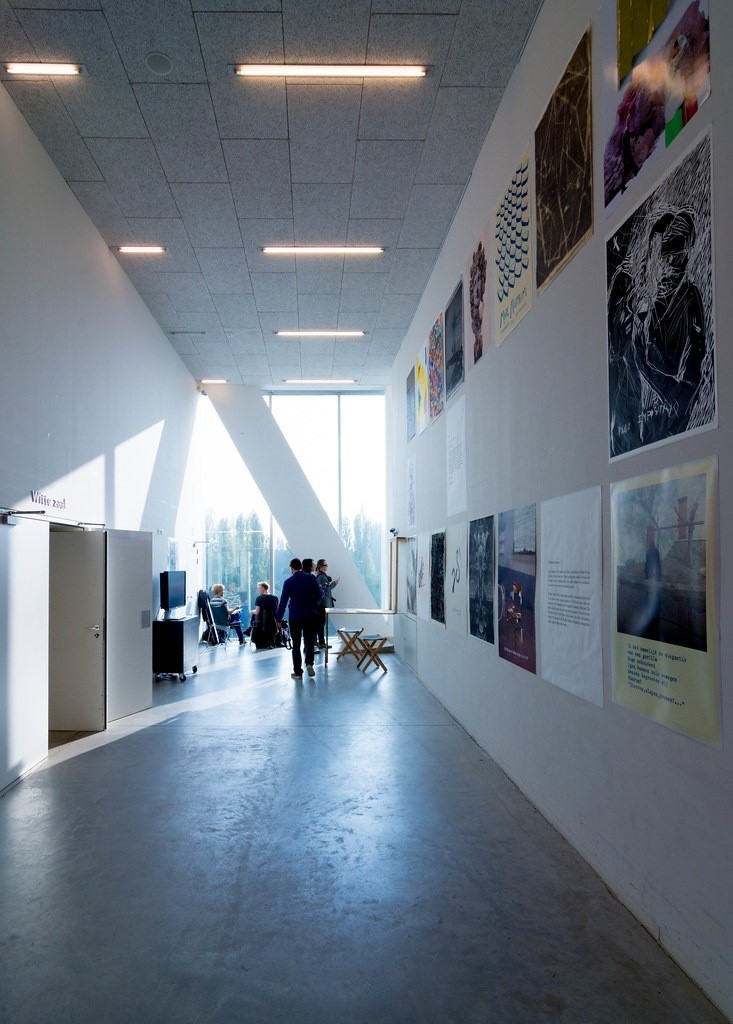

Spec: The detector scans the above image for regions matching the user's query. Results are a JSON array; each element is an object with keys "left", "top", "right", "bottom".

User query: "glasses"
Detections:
[{"left": 320, "top": 564, "right": 328, "bottom": 567}]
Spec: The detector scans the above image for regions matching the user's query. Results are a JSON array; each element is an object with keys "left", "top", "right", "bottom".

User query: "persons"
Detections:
[
  {"left": 276, "top": 558, "right": 338, "bottom": 678},
  {"left": 225, "top": 582, "right": 241, "bottom": 635},
  {"left": 242, "top": 581, "right": 279, "bottom": 636},
  {"left": 209, "top": 583, "right": 247, "bottom": 647}
]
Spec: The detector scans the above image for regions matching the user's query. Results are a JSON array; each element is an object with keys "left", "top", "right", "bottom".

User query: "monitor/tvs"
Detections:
[{"left": 160, "top": 571, "right": 187, "bottom": 620}]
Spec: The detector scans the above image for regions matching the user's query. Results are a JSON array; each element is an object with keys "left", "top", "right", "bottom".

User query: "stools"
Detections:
[
  {"left": 336, "top": 627, "right": 364, "bottom": 661},
  {"left": 356, "top": 634, "right": 387, "bottom": 672}
]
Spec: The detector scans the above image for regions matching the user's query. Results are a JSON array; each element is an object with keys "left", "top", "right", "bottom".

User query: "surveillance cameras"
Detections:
[{"left": 390, "top": 528, "right": 395, "bottom": 532}]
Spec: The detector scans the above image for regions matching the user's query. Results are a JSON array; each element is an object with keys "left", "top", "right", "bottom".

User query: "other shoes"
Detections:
[
  {"left": 240, "top": 641, "right": 246, "bottom": 646},
  {"left": 291, "top": 673, "right": 301, "bottom": 678},
  {"left": 307, "top": 664, "right": 315, "bottom": 676},
  {"left": 314, "top": 646, "right": 320, "bottom": 654},
  {"left": 318, "top": 643, "right": 332, "bottom": 649}
]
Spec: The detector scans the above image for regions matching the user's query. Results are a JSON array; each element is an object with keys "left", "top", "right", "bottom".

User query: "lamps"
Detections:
[
  {"left": 257, "top": 246, "right": 386, "bottom": 255},
  {"left": 0, "top": 62, "right": 90, "bottom": 75},
  {"left": 112, "top": 246, "right": 169, "bottom": 253},
  {"left": 228, "top": 65, "right": 435, "bottom": 78}
]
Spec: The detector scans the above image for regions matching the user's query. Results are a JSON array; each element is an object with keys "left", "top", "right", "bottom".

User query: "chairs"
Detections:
[
  {"left": 200, "top": 590, "right": 242, "bottom": 648},
  {"left": 250, "top": 605, "right": 278, "bottom": 648}
]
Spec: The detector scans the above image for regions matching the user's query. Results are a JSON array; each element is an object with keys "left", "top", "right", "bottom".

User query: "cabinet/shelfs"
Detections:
[{"left": 152, "top": 615, "right": 199, "bottom": 682}]
